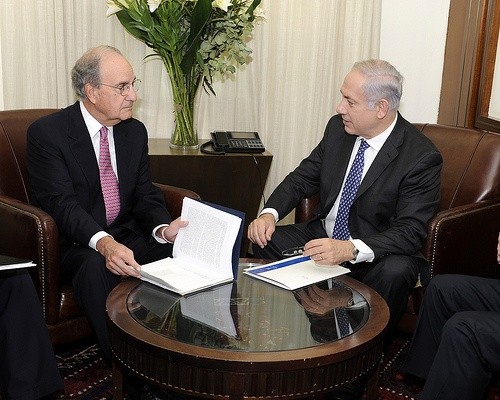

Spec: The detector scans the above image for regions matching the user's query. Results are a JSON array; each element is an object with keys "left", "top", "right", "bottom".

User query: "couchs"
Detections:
[
  {"left": 295, "top": 123, "right": 500, "bottom": 334},
  {"left": 0, "top": 110, "right": 201, "bottom": 347}
]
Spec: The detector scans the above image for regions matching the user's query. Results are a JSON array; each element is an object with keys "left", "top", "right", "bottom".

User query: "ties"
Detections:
[
  {"left": 160, "top": 309, "right": 173, "bottom": 334},
  {"left": 332, "top": 141, "right": 370, "bottom": 240},
  {"left": 332, "top": 281, "right": 350, "bottom": 338},
  {"left": 99, "top": 126, "right": 120, "bottom": 226}
]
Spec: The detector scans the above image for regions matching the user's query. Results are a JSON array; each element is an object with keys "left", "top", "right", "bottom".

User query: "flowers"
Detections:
[{"left": 104, "top": 0, "right": 268, "bottom": 146}]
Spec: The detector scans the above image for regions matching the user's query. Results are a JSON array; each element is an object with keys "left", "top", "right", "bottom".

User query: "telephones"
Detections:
[{"left": 210, "top": 131, "right": 265, "bottom": 153}]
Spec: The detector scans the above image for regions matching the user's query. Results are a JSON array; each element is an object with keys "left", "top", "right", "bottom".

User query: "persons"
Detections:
[
  {"left": 0, "top": 270, "right": 64, "bottom": 400},
  {"left": 293, "top": 280, "right": 369, "bottom": 344},
  {"left": 247, "top": 60, "right": 443, "bottom": 350},
  {"left": 379, "top": 234, "right": 500, "bottom": 400},
  {"left": 24, "top": 45, "right": 188, "bottom": 366}
]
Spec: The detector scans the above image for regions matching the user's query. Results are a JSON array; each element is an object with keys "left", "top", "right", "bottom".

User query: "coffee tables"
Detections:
[{"left": 104, "top": 257, "right": 389, "bottom": 400}]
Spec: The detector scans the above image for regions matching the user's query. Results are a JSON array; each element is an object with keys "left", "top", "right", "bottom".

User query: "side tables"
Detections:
[{"left": 147, "top": 138, "right": 273, "bottom": 257}]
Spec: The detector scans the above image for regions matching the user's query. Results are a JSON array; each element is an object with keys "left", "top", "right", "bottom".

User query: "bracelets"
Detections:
[
  {"left": 353, "top": 247, "right": 359, "bottom": 261},
  {"left": 348, "top": 298, "right": 354, "bottom": 307},
  {"left": 161, "top": 227, "right": 173, "bottom": 243}
]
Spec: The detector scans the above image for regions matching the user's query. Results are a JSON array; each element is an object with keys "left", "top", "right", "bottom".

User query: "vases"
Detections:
[{"left": 164, "top": 54, "right": 205, "bottom": 150}]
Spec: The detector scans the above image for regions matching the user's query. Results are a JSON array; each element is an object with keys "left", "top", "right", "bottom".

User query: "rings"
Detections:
[
  {"left": 316, "top": 297, "right": 320, "bottom": 302},
  {"left": 320, "top": 253, "right": 323, "bottom": 260}
]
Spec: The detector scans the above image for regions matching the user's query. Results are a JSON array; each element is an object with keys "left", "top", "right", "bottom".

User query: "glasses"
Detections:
[
  {"left": 100, "top": 79, "right": 141, "bottom": 96},
  {"left": 282, "top": 246, "right": 304, "bottom": 256}
]
